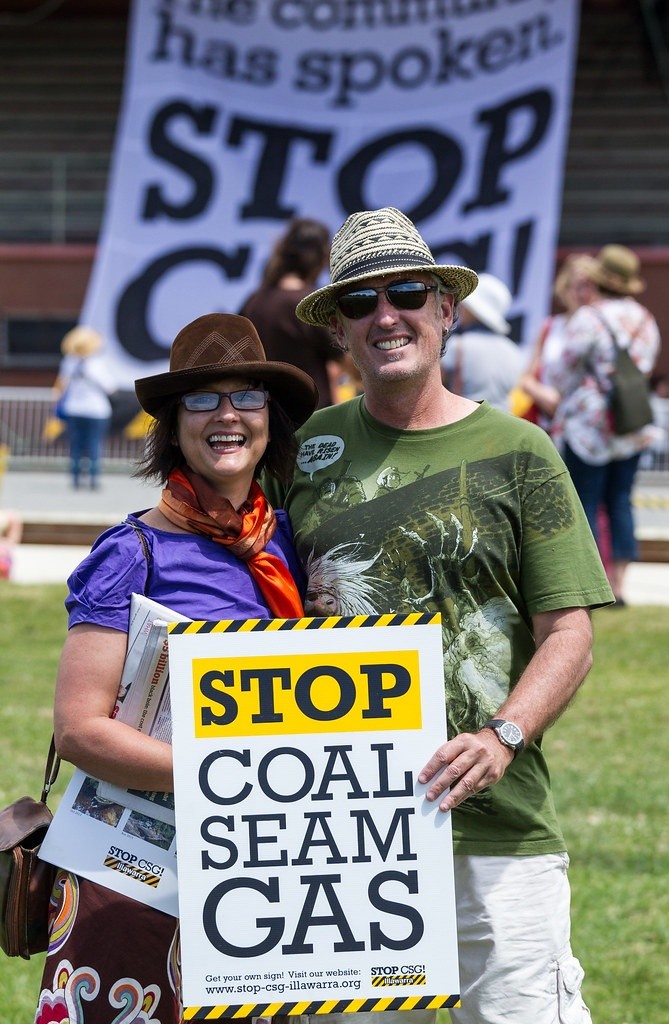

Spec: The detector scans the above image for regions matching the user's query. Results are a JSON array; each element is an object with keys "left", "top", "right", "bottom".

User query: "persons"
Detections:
[
  {"left": 237, "top": 218, "right": 668, "bottom": 608},
  {"left": 52, "top": 326, "right": 112, "bottom": 489},
  {"left": 32, "top": 311, "right": 319, "bottom": 1023},
  {"left": 273, "top": 208, "right": 617, "bottom": 1024},
  {"left": 0, "top": 512, "right": 25, "bottom": 580}
]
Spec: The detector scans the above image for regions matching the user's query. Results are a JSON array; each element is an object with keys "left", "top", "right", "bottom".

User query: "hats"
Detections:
[
  {"left": 582, "top": 242, "right": 645, "bottom": 294},
  {"left": 460, "top": 273, "right": 513, "bottom": 336},
  {"left": 60, "top": 326, "right": 100, "bottom": 357},
  {"left": 295, "top": 206, "right": 479, "bottom": 329},
  {"left": 135, "top": 313, "right": 319, "bottom": 435}
]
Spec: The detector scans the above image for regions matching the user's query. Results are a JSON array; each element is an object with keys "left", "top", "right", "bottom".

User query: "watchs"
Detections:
[{"left": 479, "top": 719, "right": 525, "bottom": 760}]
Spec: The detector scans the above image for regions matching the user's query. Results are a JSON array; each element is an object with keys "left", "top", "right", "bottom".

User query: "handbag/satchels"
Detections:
[{"left": 0, "top": 796, "right": 57, "bottom": 959}]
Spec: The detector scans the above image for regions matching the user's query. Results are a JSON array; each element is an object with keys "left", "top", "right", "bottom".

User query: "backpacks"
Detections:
[{"left": 581, "top": 304, "right": 654, "bottom": 434}]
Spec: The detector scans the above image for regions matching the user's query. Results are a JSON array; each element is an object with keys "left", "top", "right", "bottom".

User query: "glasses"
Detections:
[
  {"left": 333, "top": 280, "right": 436, "bottom": 321},
  {"left": 175, "top": 388, "right": 270, "bottom": 412}
]
